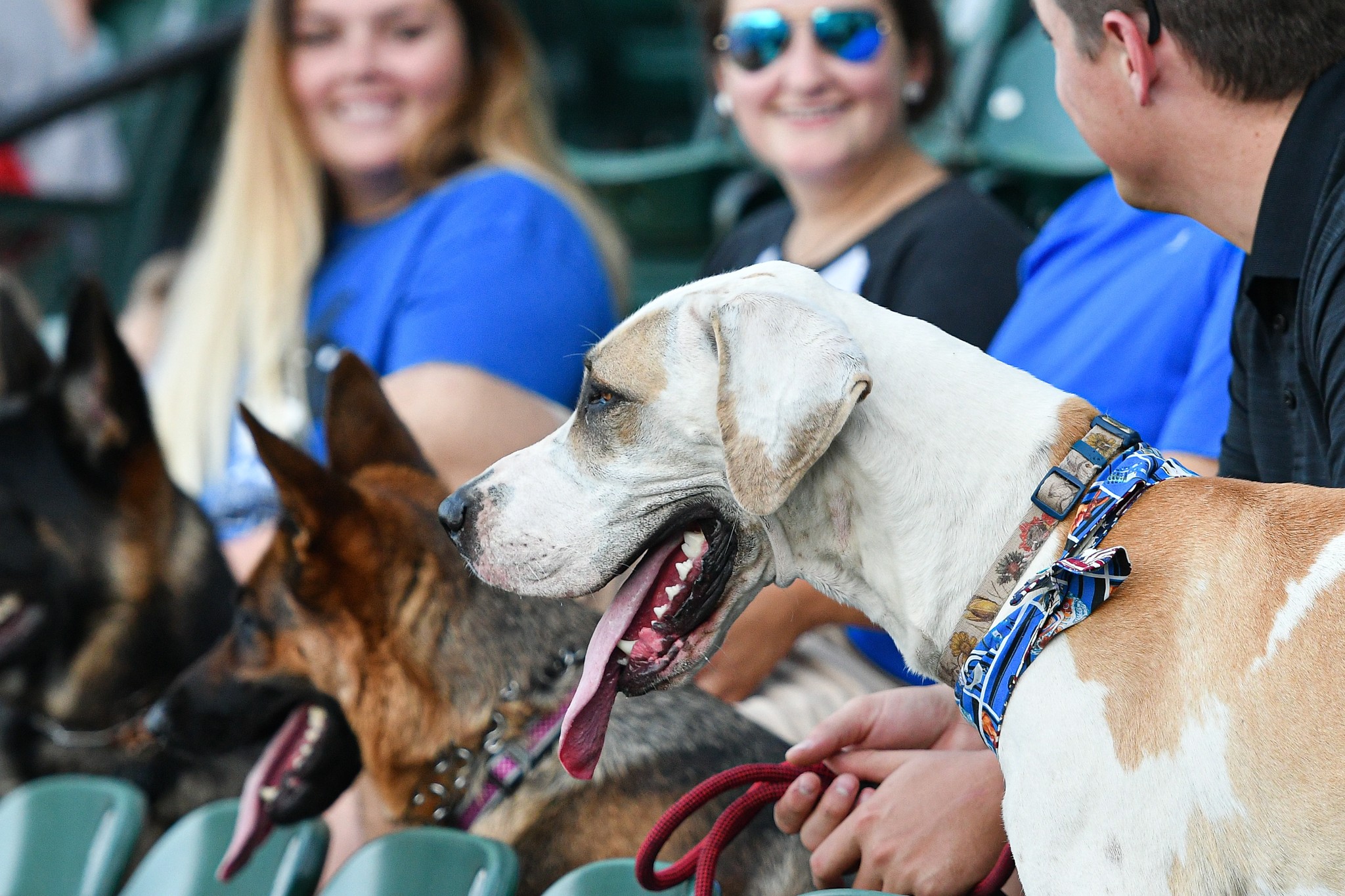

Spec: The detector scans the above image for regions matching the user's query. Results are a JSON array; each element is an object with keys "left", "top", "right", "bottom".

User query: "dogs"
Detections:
[
  {"left": 437, "top": 260, "right": 1345, "bottom": 896},
  {"left": 0, "top": 274, "right": 271, "bottom": 896},
  {"left": 140, "top": 345, "right": 858, "bottom": 896}
]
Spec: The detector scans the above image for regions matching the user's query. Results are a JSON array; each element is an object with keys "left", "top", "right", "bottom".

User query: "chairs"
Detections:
[
  {"left": 0, "top": 773, "right": 148, "bottom": 896},
  {"left": 543, "top": 856, "right": 721, "bottom": 896},
  {"left": 118, "top": 797, "right": 331, "bottom": 896},
  {"left": 558, "top": 0, "right": 1111, "bottom": 245},
  {"left": 320, "top": 826, "right": 520, "bottom": 895}
]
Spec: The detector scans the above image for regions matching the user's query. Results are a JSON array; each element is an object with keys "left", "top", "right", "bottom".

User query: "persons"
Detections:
[
  {"left": 315, "top": 0, "right": 1344, "bottom": 894},
  {"left": 113, "top": 1, "right": 628, "bottom": 588}
]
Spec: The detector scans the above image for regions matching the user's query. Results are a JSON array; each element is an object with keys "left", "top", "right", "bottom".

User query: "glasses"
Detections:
[{"left": 717, "top": 8, "right": 905, "bottom": 71}]
